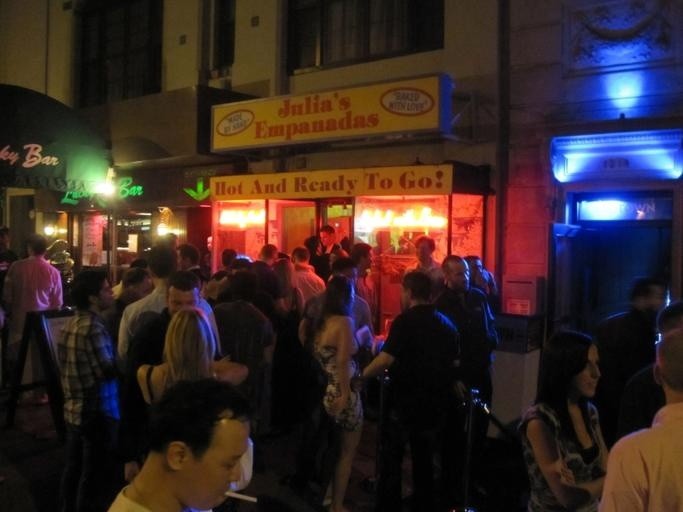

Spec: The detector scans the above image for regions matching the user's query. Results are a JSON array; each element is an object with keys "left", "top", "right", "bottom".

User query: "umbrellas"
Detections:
[{"left": 0, "top": 83, "right": 118, "bottom": 193}]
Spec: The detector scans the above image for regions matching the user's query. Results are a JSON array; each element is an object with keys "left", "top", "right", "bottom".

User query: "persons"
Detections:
[{"left": 0, "top": 226, "right": 683, "bottom": 512}]
